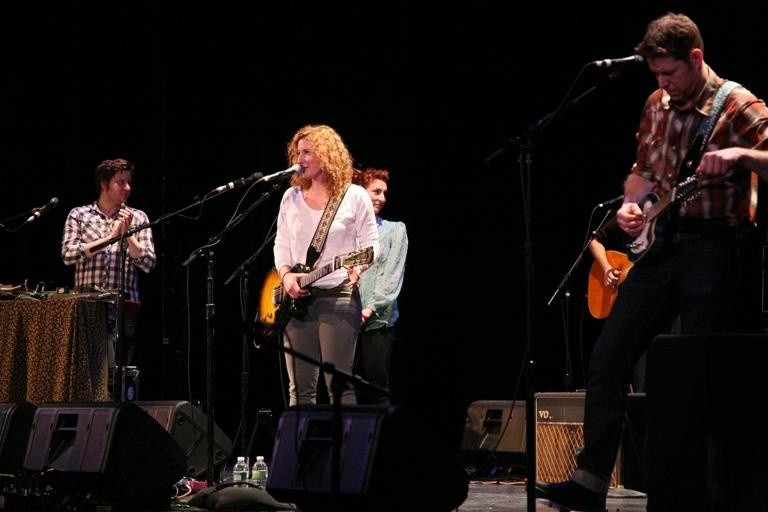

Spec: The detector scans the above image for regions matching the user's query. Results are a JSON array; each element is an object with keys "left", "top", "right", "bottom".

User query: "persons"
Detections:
[
  {"left": 525, "top": 13, "right": 768, "bottom": 512},
  {"left": 348, "top": 163, "right": 417, "bottom": 415},
  {"left": 273, "top": 120, "right": 381, "bottom": 407},
  {"left": 60, "top": 158, "right": 158, "bottom": 395}
]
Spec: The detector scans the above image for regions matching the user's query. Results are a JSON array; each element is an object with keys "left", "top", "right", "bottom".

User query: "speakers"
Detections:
[
  {"left": 1, "top": 399, "right": 234, "bottom": 512},
  {"left": 266, "top": 391, "right": 647, "bottom": 511}
]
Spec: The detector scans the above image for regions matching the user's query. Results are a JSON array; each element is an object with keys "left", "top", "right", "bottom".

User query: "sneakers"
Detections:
[{"left": 535, "top": 481, "right": 607, "bottom": 512}]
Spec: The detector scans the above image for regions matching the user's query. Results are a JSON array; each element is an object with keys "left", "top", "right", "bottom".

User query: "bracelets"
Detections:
[{"left": 282, "top": 272, "right": 290, "bottom": 279}]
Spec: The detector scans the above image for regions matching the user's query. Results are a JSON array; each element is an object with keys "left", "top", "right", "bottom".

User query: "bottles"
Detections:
[
  {"left": 252, "top": 456, "right": 268, "bottom": 491},
  {"left": 233, "top": 456, "right": 249, "bottom": 489}
]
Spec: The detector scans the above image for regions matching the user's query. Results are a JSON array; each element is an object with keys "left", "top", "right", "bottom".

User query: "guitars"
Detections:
[
  {"left": 259, "top": 246, "right": 374, "bottom": 326},
  {"left": 628, "top": 173, "right": 700, "bottom": 261},
  {"left": 588, "top": 251, "right": 636, "bottom": 318}
]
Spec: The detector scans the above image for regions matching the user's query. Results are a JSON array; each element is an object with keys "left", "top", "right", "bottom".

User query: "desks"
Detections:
[{"left": 1, "top": 290, "right": 108, "bottom": 404}]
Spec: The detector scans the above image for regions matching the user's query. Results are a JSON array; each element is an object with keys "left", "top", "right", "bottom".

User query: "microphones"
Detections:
[
  {"left": 27, "top": 196, "right": 59, "bottom": 221},
  {"left": 209, "top": 171, "right": 263, "bottom": 193},
  {"left": 265, "top": 163, "right": 302, "bottom": 183},
  {"left": 598, "top": 194, "right": 625, "bottom": 208}
]
